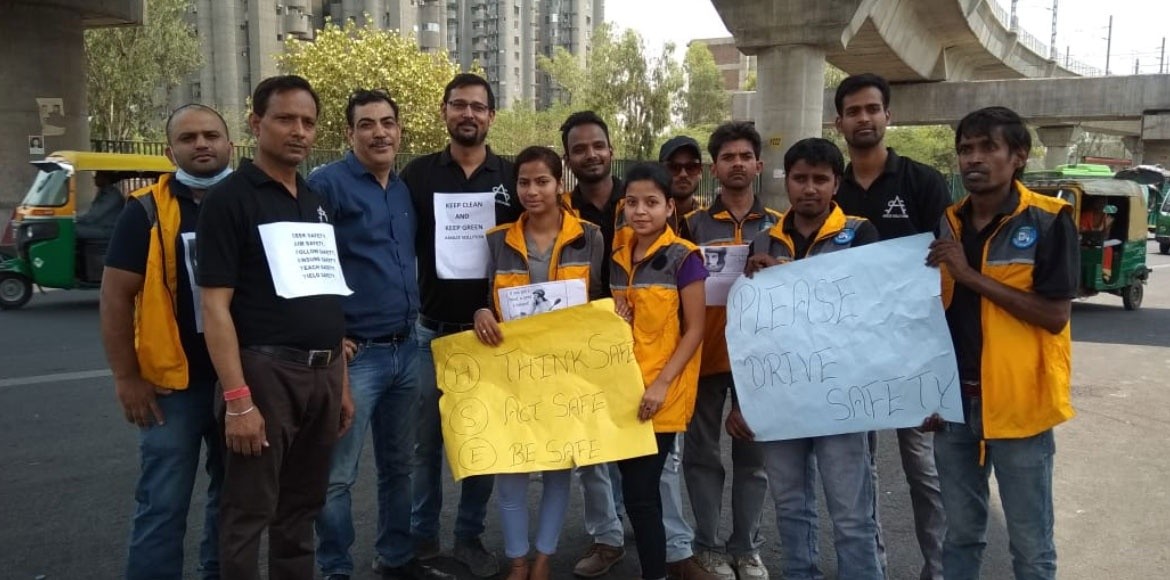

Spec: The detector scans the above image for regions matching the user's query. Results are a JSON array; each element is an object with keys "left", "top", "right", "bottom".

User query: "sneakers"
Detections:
[
  {"left": 695, "top": 549, "right": 736, "bottom": 580},
  {"left": 733, "top": 553, "right": 769, "bottom": 580}
]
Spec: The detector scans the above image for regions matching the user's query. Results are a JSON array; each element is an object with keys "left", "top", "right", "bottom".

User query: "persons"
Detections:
[
  {"left": 97, "top": 104, "right": 235, "bottom": 580},
  {"left": 560, "top": 109, "right": 712, "bottom": 580},
  {"left": 608, "top": 164, "right": 710, "bottom": 580},
  {"left": 306, "top": 89, "right": 421, "bottom": 580},
  {"left": 924, "top": 107, "right": 1075, "bottom": 580},
  {"left": 193, "top": 75, "right": 354, "bottom": 580},
  {"left": 725, "top": 137, "right": 888, "bottom": 580},
  {"left": 679, "top": 119, "right": 784, "bottom": 580},
  {"left": 76, "top": 170, "right": 126, "bottom": 238},
  {"left": 473, "top": 146, "right": 604, "bottom": 580},
  {"left": 658, "top": 134, "right": 702, "bottom": 240},
  {"left": 398, "top": 74, "right": 525, "bottom": 577},
  {"left": 831, "top": 73, "right": 956, "bottom": 580}
]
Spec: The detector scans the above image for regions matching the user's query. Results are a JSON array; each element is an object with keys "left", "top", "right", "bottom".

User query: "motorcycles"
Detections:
[
  {"left": 1026, "top": 177, "right": 1153, "bottom": 312},
  {"left": 0, "top": 151, "right": 178, "bottom": 312},
  {"left": 1112, "top": 164, "right": 1170, "bottom": 256}
]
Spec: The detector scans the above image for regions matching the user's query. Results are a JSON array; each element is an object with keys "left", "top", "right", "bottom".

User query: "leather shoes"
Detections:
[
  {"left": 666, "top": 556, "right": 722, "bottom": 580},
  {"left": 573, "top": 543, "right": 626, "bottom": 575}
]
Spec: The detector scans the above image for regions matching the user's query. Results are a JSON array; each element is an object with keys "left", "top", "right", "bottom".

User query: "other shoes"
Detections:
[
  {"left": 420, "top": 556, "right": 465, "bottom": 580},
  {"left": 530, "top": 552, "right": 554, "bottom": 580},
  {"left": 322, "top": 574, "right": 350, "bottom": 580},
  {"left": 380, "top": 560, "right": 425, "bottom": 580},
  {"left": 507, "top": 554, "right": 530, "bottom": 580},
  {"left": 453, "top": 537, "right": 500, "bottom": 575}
]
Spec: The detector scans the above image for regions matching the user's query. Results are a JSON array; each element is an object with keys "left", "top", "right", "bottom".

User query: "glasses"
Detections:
[
  {"left": 663, "top": 161, "right": 702, "bottom": 176},
  {"left": 445, "top": 98, "right": 491, "bottom": 112}
]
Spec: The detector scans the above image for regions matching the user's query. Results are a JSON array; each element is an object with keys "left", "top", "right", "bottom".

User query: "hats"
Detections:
[{"left": 658, "top": 135, "right": 701, "bottom": 166}]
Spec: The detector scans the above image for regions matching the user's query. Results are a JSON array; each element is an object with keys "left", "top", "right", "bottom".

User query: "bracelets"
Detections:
[
  {"left": 224, "top": 386, "right": 250, "bottom": 401},
  {"left": 226, "top": 406, "right": 253, "bottom": 416}
]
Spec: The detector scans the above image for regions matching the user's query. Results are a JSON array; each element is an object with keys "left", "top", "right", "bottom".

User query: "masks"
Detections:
[{"left": 169, "top": 145, "right": 233, "bottom": 188}]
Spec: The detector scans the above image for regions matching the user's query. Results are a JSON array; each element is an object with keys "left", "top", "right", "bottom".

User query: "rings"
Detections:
[
  {"left": 481, "top": 330, "right": 486, "bottom": 333},
  {"left": 645, "top": 407, "right": 650, "bottom": 410}
]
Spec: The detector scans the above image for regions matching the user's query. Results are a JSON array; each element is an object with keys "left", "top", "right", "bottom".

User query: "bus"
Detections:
[{"left": 1023, "top": 164, "right": 1114, "bottom": 182}]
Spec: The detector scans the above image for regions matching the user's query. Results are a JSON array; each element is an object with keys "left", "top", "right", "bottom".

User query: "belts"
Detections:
[
  {"left": 960, "top": 380, "right": 982, "bottom": 397},
  {"left": 249, "top": 343, "right": 343, "bottom": 367},
  {"left": 356, "top": 328, "right": 414, "bottom": 345},
  {"left": 419, "top": 316, "right": 476, "bottom": 334}
]
